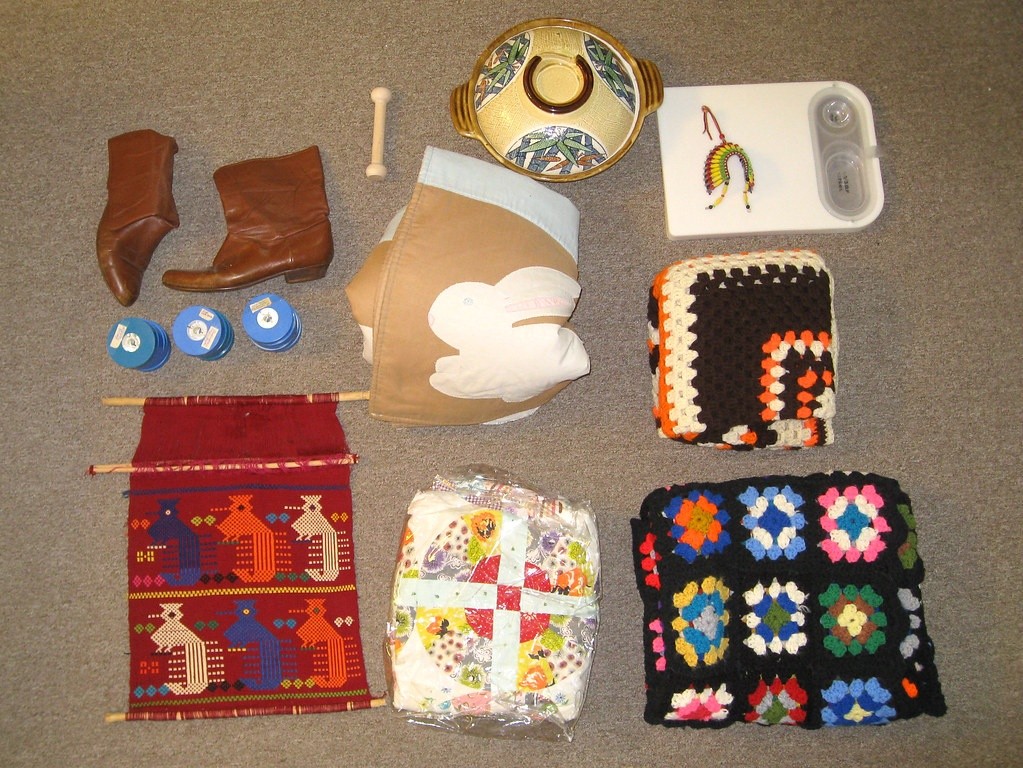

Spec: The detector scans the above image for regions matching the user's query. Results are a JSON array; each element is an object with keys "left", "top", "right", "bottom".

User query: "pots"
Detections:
[{"left": 450, "top": 18, "right": 664, "bottom": 183}]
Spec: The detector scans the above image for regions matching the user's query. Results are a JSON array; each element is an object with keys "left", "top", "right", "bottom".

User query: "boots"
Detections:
[
  {"left": 161, "top": 147, "right": 334, "bottom": 293},
  {"left": 95, "top": 128, "right": 179, "bottom": 307}
]
